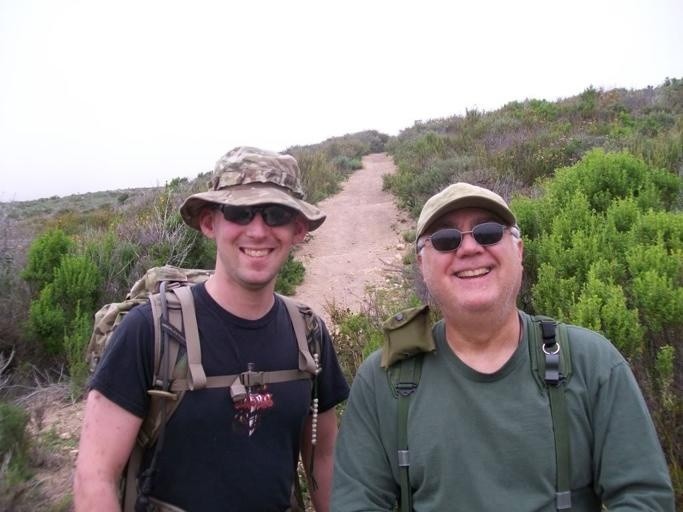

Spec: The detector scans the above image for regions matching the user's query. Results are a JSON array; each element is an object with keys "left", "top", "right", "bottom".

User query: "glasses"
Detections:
[
  {"left": 420, "top": 223, "right": 518, "bottom": 252},
  {"left": 206, "top": 204, "right": 298, "bottom": 226}
]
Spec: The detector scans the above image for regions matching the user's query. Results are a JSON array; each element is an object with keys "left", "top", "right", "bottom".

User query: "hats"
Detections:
[
  {"left": 179, "top": 146, "right": 327, "bottom": 232},
  {"left": 415, "top": 182, "right": 519, "bottom": 254}
]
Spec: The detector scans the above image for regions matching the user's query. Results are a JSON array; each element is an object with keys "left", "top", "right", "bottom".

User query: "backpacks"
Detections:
[{"left": 85, "top": 264, "right": 322, "bottom": 480}]
